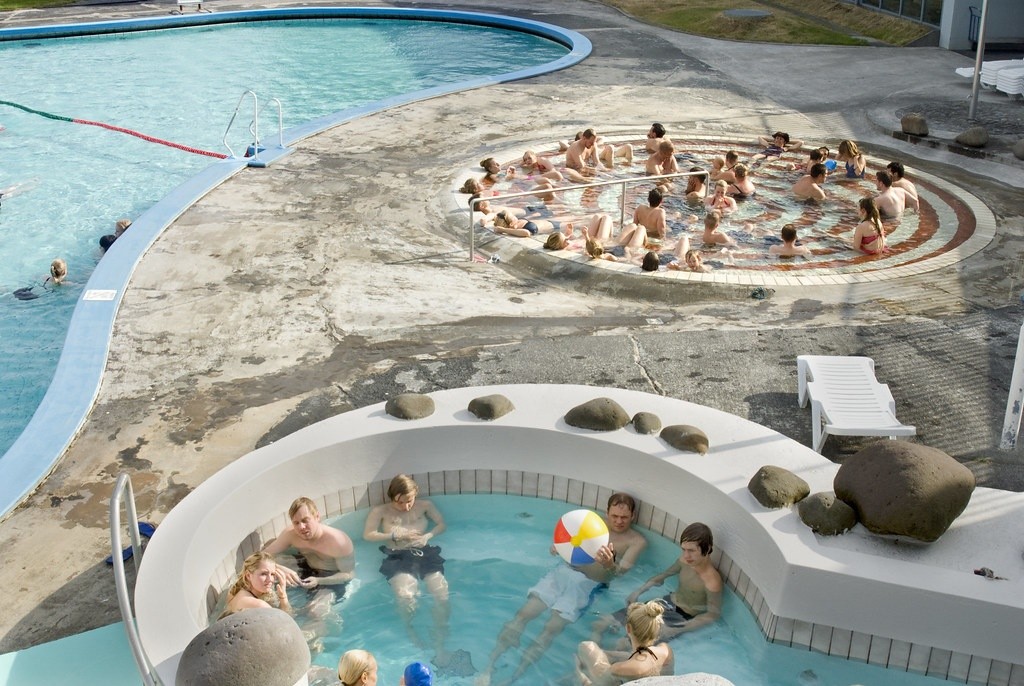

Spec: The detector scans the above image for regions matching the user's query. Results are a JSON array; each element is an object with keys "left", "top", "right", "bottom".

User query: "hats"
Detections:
[{"left": 404, "top": 662, "right": 432, "bottom": 686}]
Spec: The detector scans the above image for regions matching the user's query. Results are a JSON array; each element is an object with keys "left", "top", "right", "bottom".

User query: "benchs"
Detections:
[
  {"left": 797, "top": 353, "right": 918, "bottom": 457},
  {"left": 956, "top": 58, "right": 1024, "bottom": 99}
]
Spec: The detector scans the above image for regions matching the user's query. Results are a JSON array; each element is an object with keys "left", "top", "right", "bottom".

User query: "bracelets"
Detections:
[{"left": 392, "top": 532, "right": 396, "bottom": 544}]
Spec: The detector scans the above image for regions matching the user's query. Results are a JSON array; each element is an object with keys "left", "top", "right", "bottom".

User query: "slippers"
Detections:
[
  {"left": 137, "top": 522, "right": 156, "bottom": 537},
  {"left": 106, "top": 546, "right": 133, "bottom": 562}
]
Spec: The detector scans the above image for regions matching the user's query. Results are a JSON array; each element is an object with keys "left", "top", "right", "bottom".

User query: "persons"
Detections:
[
  {"left": 401, "top": 662, "right": 433, "bottom": 686},
  {"left": 114, "top": 220, "right": 132, "bottom": 238},
  {"left": 572, "top": 601, "right": 674, "bottom": 686},
  {"left": 99, "top": 234, "right": 117, "bottom": 257},
  {"left": 0, "top": 175, "right": 42, "bottom": 203},
  {"left": 474, "top": 493, "right": 647, "bottom": 686},
  {"left": 457, "top": 123, "right": 920, "bottom": 272},
  {"left": 0, "top": 259, "right": 86, "bottom": 303},
  {"left": 226, "top": 551, "right": 327, "bottom": 645},
  {"left": 363, "top": 473, "right": 450, "bottom": 668},
  {"left": 262, "top": 497, "right": 356, "bottom": 635},
  {"left": 337, "top": 649, "right": 377, "bottom": 686},
  {"left": 590, "top": 522, "right": 721, "bottom": 661}
]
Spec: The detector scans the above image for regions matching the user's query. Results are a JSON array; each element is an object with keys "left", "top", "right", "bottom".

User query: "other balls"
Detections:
[
  {"left": 824, "top": 160, "right": 838, "bottom": 171},
  {"left": 552, "top": 508, "right": 611, "bottom": 567}
]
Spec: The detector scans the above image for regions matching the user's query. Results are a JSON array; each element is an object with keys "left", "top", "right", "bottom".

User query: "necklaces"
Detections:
[{"left": 242, "top": 586, "right": 259, "bottom": 599}]
